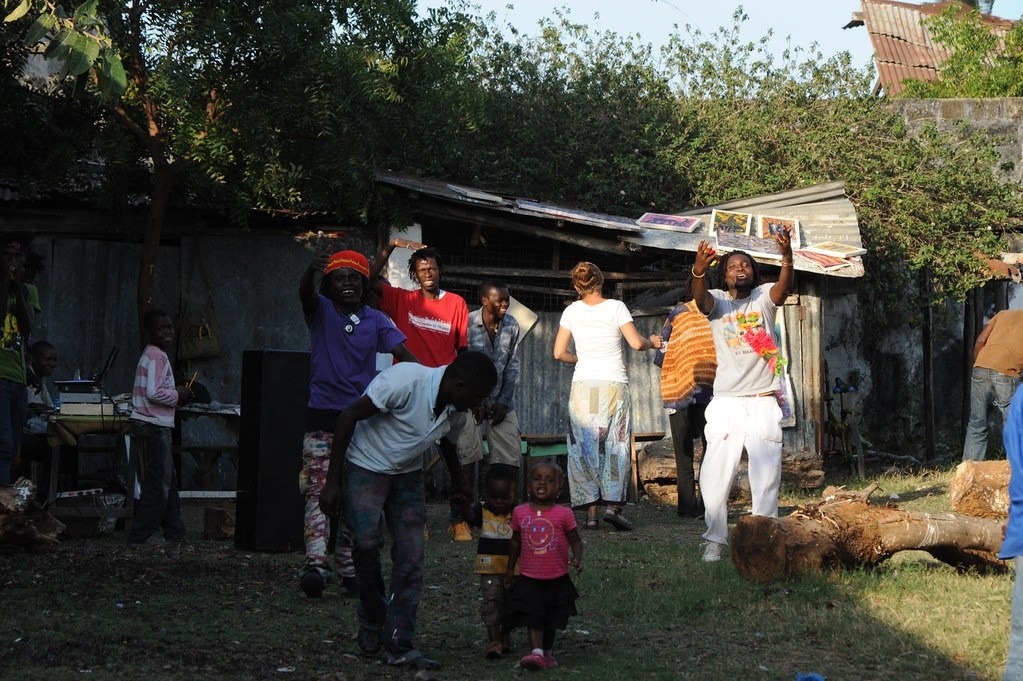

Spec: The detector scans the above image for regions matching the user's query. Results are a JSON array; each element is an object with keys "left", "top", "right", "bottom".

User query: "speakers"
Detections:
[{"left": 233, "top": 349, "right": 313, "bottom": 553}]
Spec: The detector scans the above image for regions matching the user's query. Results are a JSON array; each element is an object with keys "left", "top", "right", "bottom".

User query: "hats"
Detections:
[{"left": 321, "top": 250, "right": 370, "bottom": 279}]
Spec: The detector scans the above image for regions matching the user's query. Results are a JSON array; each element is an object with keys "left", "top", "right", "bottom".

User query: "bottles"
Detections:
[{"left": 73, "top": 368, "right": 81, "bottom": 380}]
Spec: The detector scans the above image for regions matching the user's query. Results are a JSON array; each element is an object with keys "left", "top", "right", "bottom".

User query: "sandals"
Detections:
[
  {"left": 584, "top": 517, "right": 600, "bottom": 529},
  {"left": 604, "top": 506, "right": 635, "bottom": 530}
]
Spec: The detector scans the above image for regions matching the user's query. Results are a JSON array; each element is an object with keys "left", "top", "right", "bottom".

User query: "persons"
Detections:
[
  {"left": 553, "top": 261, "right": 665, "bottom": 531},
  {"left": 298, "top": 245, "right": 421, "bottom": 598},
  {"left": 462, "top": 463, "right": 521, "bottom": 660},
  {"left": 654, "top": 272, "right": 717, "bottom": 518},
  {"left": 363, "top": 236, "right": 471, "bottom": 541},
  {"left": 962, "top": 309, "right": 1023, "bottom": 462},
  {"left": 318, "top": 351, "right": 498, "bottom": 668},
  {"left": 127, "top": 310, "right": 200, "bottom": 550},
  {"left": 998, "top": 385, "right": 1023, "bottom": 680},
  {"left": 503, "top": 461, "right": 583, "bottom": 670},
  {"left": 441, "top": 278, "right": 521, "bottom": 500},
  {"left": 0, "top": 242, "right": 66, "bottom": 546},
  {"left": 692, "top": 224, "right": 794, "bottom": 561}
]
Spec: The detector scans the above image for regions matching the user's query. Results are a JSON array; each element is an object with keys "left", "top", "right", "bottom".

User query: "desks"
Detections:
[
  {"left": 46, "top": 414, "right": 145, "bottom": 533},
  {"left": 477, "top": 431, "right": 667, "bottom": 505}
]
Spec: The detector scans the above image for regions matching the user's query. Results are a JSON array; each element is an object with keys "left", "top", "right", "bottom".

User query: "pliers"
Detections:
[{"left": 199, "top": 316, "right": 211, "bottom": 341}]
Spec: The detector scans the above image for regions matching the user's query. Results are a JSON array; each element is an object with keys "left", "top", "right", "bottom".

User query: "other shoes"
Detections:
[
  {"left": 302, "top": 573, "right": 323, "bottom": 598},
  {"left": 487, "top": 641, "right": 502, "bottom": 657},
  {"left": 544, "top": 653, "right": 558, "bottom": 667},
  {"left": 342, "top": 577, "right": 360, "bottom": 592},
  {"left": 133, "top": 536, "right": 165, "bottom": 552},
  {"left": 520, "top": 652, "right": 546, "bottom": 670},
  {"left": 500, "top": 634, "right": 512, "bottom": 652}
]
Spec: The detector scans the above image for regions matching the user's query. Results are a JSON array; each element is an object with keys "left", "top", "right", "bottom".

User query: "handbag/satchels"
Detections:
[{"left": 177, "top": 301, "right": 221, "bottom": 359}]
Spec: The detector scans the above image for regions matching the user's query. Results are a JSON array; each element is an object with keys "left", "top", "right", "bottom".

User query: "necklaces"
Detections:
[{"left": 331, "top": 298, "right": 364, "bottom": 333}]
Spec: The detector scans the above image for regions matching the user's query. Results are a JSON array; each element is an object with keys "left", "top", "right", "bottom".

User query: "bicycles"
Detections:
[{"left": 824, "top": 342, "right": 865, "bottom": 477}]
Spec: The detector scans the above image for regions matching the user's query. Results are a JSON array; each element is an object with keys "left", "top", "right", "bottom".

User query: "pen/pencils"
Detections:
[{"left": 186, "top": 371, "right": 198, "bottom": 390}]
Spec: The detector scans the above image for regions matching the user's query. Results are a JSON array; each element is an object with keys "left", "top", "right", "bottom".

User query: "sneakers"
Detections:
[
  {"left": 448, "top": 522, "right": 474, "bottom": 541},
  {"left": 358, "top": 627, "right": 384, "bottom": 654},
  {"left": 423, "top": 524, "right": 430, "bottom": 541},
  {"left": 701, "top": 542, "right": 723, "bottom": 561},
  {"left": 409, "top": 655, "right": 442, "bottom": 670}
]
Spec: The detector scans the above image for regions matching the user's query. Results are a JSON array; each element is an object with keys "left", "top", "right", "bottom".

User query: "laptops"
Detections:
[{"left": 53, "top": 346, "right": 119, "bottom": 385}]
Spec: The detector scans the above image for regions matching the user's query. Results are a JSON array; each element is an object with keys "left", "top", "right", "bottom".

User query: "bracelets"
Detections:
[
  {"left": 692, "top": 266, "right": 705, "bottom": 278},
  {"left": 780, "top": 257, "right": 795, "bottom": 266}
]
